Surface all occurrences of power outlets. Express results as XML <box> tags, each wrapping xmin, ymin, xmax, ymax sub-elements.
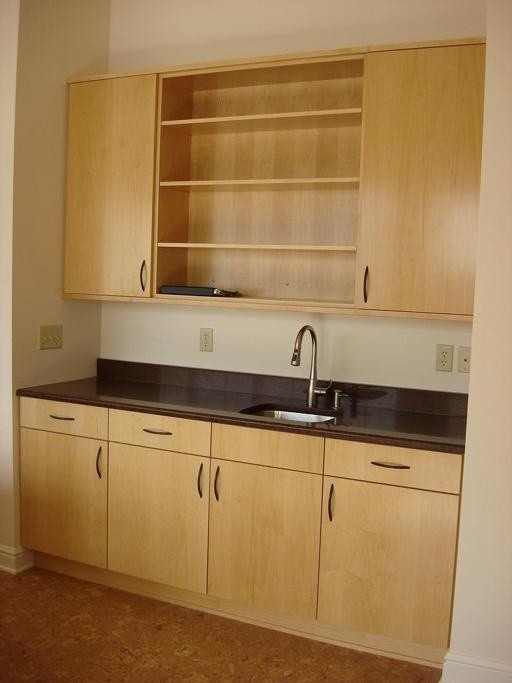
<box><xmin>199</xmin><ymin>327</ymin><xmax>213</xmax><ymax>352</ymax></box>
<box><xmin>435</xmin><ymin>343</ymin><xmax>454</xmax><ymax>372</ymax></box>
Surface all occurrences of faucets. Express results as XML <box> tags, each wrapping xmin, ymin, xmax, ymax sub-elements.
<box><xmin>290</xmin><ymin>324</ymin><xmax>319</xmax><ymax>408</ymax></box>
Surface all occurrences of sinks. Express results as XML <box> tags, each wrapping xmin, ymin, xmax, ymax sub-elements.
<box><xmin>238</xmin><ymin>402</ymin><xmax>338</xmax><ymax>424</ymax></box>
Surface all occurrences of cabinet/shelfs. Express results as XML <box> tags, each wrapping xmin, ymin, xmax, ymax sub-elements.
<box><xmin>359</xmin><ymin>37</ymin><xmax>484</xmax><ymax>315</ymax></box>
<box><xmin>157</xmin><ymin>49</ymin><xmax>365</xmax><ymax>308</ymax></box>
<box><xmin>18</xmin><ymin>395</ymin><xmax>109</xmax><ymax>572</ymax></box>
<box><xmin>58</xmin><ymin>69</ymin><xmax>192</xmax><ymax>304</ymax></box>
<box><xmin>107</xmin><ymin>407</ymin><xmax>326</xmax><ymax>629</ymax></box>
<box><xmin>315</xmin><ymin>434</ymin><xmax>464</xmax><ymax>651</ymax></box>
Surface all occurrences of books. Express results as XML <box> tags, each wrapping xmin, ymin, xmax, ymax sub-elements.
<box><xmin>160</xmin><ymin>285</ymin><xmax>240</xmax><ymax>296</ymax></box>
